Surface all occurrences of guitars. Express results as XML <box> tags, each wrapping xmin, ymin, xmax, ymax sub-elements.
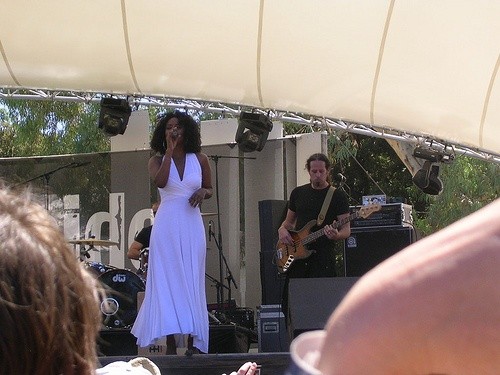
<box><xmin>275</xmin><ymin>199</ymin><xmax>383</xmax><ymax>274</ymax></box>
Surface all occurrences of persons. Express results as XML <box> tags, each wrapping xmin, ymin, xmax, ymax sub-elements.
<box><xmin>131</xmin><ymin>110</ymin><xmax>214</xmax><ymax>353</ymax></box>
<box><xmin>222</xmin><ymin>362</ymin><xmax>257</xmax><ymax>375</ymax></box>
<box><xmin>290</xmin><ymin>200</ymin><xmax>500</xmax><ymax>375</ymax></box>
<box><xmin>278</xmin><ymin>153</ymin><xmax>350</xmax><ymax>321</ymax></box>
<box><xmin>0</xmin><ymin>190</ymin><xmax>160</xmax><ymax>375</ymax></box>
<box><xmin>127</xmin><ymin>200</ymin><xmax>161</xmax><ymax>262</ymax></box>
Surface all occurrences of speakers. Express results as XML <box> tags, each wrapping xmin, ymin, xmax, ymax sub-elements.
<box><xmin>208</xmin><ymin>324</ymin><xmax>253</xmax><ymax>355</ymax></box>
<box><xmin>95</xmin><ymin>329</ymin><xmax>138</xmax><ymax>357</ymax></box>
<box><xmin>344</xmin><ymin>226</ymin><xmax>420</xmax><ymax>280</ymax></box>
<box><xmin>286</xmin><ymin>277</ymin><xmax>360</xmax><ymax>338</ymax></box>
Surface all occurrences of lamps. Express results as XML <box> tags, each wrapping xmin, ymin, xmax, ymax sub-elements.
<box><xmin>236</xmin><ymin>111</ymin><xmax>273</xmax><ymax>153</ymax></box>
<box><xmin>98</xmin><ymin>98</ymin><xmax>132</xmax><ymax>137</ymax></box>
<box><xmin>413</xmin><ymin>148</ymin><xmax>454</xmax><ymax>195</ymax></box>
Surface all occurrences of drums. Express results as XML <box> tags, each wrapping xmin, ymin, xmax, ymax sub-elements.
<box><xmin>139</xmin><ymin>247</ymin><xmax>149</xmax><ymax>276</ymax></box>
<box><xmin>91</xmin><ymin>266</ymin><xmax>145</xmax><ymax>330</ymax></box>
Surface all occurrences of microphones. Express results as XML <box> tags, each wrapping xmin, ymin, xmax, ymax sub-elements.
<box><xmin>209</xmin><ymin>221</ymin><xmax>212</xmax><ymax>242</ymax></box>
<box><xmin>169</xmin><ymin>129</ymin><xmax>178</xmax><ymax>140</ymax></box>
<box><xmin>70</xmin><ymin>161</ymin><xmax>92</xmax><ymax>168</ymax></box>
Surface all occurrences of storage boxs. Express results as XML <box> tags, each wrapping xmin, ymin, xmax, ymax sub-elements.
<box><xmin>256</xmin><ymin>304</ymin><xmax>290</xmax><ymax>353</ymax></box>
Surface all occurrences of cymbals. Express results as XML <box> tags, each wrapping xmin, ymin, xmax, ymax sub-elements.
<box><xmin>201</xmin><ymin>212</ymin><xmax>223</xmax><ymax>216</ymax></box>
<box><xmin>67</xmin><ymin>239</ymin><xmax>119</xmax><ymax>246</ymax></box>
